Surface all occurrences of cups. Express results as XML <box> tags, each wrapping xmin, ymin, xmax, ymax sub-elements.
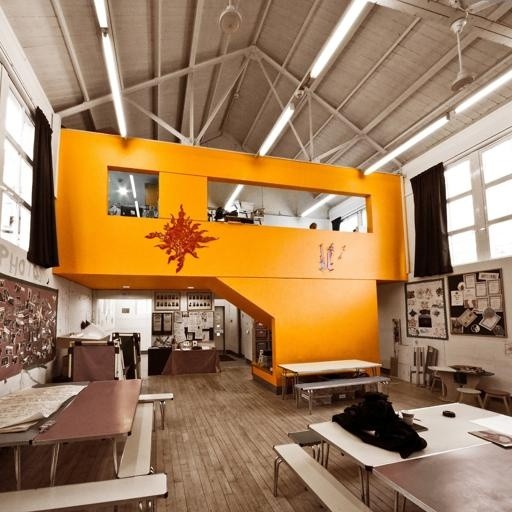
<box><xmin>402</xmin><ymin>410</ymin><xmax>415</xmax><ymax>427</ymax></box>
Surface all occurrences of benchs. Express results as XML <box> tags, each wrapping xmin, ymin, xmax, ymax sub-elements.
<box><xmin>274</xmin><ymin>427</ymin><xmax>378</xmax><ymax>512</ymax></box>
<box><xmin>0</xmin><ymin>393</ymin><xmax>173</xmax><ymax>511</ymax></box>
<box><xmin>279</xmin><ymin>369</ymin><xmax>392</xmax><ymax>415</ymax></box>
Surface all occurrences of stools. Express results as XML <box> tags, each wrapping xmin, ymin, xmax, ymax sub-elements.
<box><xmin>455</xmin><ymin>388</ymin><xmax>510</xmax><ymax>413</ymax></box>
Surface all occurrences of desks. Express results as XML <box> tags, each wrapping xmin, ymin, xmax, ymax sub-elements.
<box><xmin>276</xmin><ymin>358</ymin><xmax>383</xmax><ymax>400</ymax></box>
<box><xmin>305</xmin><ymin>400</ymin><xmax>512</xmax><ymax>512</ymax></box>
<box><xmin>428</xmin><ymin>365</ymin><xmax>484</xmax><ymax>402</ymax></box>
<box><xmin>0</xmin><ymin>378</ymin><xmax>144</xmax><ymax>511</ymax></box>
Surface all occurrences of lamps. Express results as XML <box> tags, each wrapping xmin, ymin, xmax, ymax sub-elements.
<box><xmin>309</xmin><ymin>0</ymin><xmax>371</xmax><ymax>79</ymax></box>
<box><xmin>299</xmin><ymin>194</ymin><xmax>337</xmax><ymax>218</ymax></box>
<box><xmin>454</xmin><ymin>69</ymin><xmax>511</xmax><ymax>114</ymax></box>
<box><xmin>220</xmin><ymin>0</ymin><xmax>242</xmax><ymax>35</ymax></box>
<box><xmin>223</xmin><ymin>184</ymin><xmax>245</xmax><ymax>212</ymax></box>
<box><xmin>448</xmin><ymin>13</ymin><xmax>475</xmax><ymax>93</ymax></box>
<box><xmin>257</xmin><ymin>102</ymin><xmax>296</xmax><ymax>157</ymax></box>
<box><xmin>363</xmin><ymin>112</ymin><xmax>451</xmax><ymax>178</ymax></box>
<box><xmin>92</xmin><ymin>0</ymin><xmax>142</xmax><ymax>220</ymax></box>
